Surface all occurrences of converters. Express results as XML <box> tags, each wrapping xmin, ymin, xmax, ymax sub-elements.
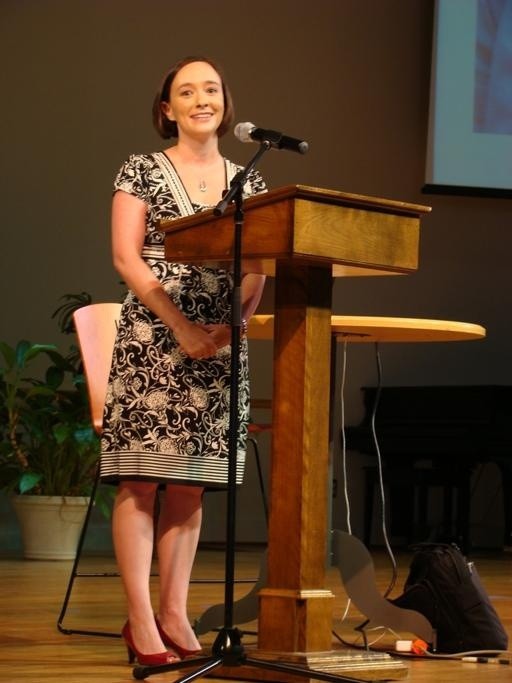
<box><xmin>395</xmin><ymin>641</ymin><xmax>412</xmax><ymax>652</ymax></box>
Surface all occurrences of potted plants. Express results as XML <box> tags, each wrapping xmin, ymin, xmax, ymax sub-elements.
<box><xmin>1</xmin><ymin>293</ymin><xmax>119</xmax><ymax>564</ymax></box>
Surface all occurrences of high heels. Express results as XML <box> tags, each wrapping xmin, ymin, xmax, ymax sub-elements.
<box><xmin>122</xmin><ymin>614</ymin><xmax>208</xmax><ymax>666</ymax></box>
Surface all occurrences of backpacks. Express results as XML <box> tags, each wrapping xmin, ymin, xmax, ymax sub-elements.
<box><xmin>386</xmin><ymin>542</ymin><xmax>509</xmax><ymax>657</ymax></box>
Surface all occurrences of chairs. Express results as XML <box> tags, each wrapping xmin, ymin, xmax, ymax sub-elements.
<box><xmin>56</xmin><ymin>301</ymin><xmax>270</xmax><ymax>638</ymax></box>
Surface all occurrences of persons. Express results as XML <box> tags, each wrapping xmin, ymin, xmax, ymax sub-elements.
<box><xmin>102</xmin><ymin>58</ymin><xmax>271</xmax><ymax>665</ymax></box>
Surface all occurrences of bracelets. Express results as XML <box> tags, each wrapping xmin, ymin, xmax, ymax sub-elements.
<box><xmin>241</xmin><ymin>319</ymin><xmax>248</xmax><ymax>334</ymax></box>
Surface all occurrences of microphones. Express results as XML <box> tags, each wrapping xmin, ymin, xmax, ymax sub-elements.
<box><xmin>233</xmin><ymin>121</ymin><xmax>309</xmax><ymax>156</ymax></box>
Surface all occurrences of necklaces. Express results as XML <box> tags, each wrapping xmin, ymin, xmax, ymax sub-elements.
<box><xmin>199</xmin><ymin>178</ymin><xmax>208</xmax><ymax>192</ymax></box>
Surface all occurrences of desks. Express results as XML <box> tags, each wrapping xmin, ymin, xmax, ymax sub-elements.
<box><xmin>245</xmin><ymin>314</ymin><xmax>488</xmax><ymax>651</ymax></box>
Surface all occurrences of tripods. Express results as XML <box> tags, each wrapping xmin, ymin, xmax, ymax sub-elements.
<box><xmin>132</xmin><ymin>139</ymin><xmax>377</xmax><ymax>683</ymax></box>
